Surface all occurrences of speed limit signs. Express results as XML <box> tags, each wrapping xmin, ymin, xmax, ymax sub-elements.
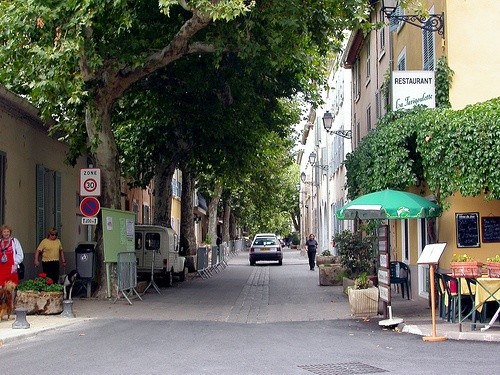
<box><xmin>84</xmin><ymin>178</ymin><xmax>98</xmax><ymax>193</ymax></box>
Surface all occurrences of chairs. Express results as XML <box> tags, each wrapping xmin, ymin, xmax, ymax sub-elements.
<box><xmin>390</xmin><ymin>260</ymin><xmax>410</xmax><ymax>300</ymax></box>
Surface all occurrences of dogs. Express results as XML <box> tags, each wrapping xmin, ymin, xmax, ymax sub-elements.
<box><xmin>0</xmin><ymin>281</ymin><xmax>16</xmax><ymax>321</ymax></box>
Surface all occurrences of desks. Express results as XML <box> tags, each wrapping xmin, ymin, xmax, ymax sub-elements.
<box><xmin>438</xmin><ymin>273</ymin><xmax>500</xmax><ymax>330</ymax></box>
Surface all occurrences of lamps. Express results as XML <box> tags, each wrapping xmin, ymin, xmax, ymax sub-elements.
<box><xmin>292</xmin><ymin>195</ymin><xmax>303</xmax><ymax>204</ymax></box>
<box><xmin>296</xmin><ymin>182</ymin><xmax>307</xmax><ymax>195</ymax></box>
<box><xmin>309</xmin><ymin>152</ymin><xmax>327</xmax><ymax>171</ymax></box>
<box><xmin>381</xmin><ymin>0</ymin><xmax>444</xmax><ymax>38</ymax></box>
<box><xmin>322</xmin><ymin>111</ymin><xmax>351</xmax><ymax>139</ymax></box>
<box><xmin>301</xmin><ymin>172</ymin><xmax>313</xmax><ymax>186</ymax></box>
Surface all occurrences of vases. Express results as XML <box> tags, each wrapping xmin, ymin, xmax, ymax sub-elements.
<box><xmin>450</xmin><ymin>261</ymin><xmax>484</xmax><ymax>276</ymax></box>
<box><xmin>15</xmin><ymin>290</ymin><xmax>64</xmax><ymax>314</ymax></box>
<box><xmin>486</xmin><ymin>261</ymin><xmax>500</xmax><ymax>277</ymax></box>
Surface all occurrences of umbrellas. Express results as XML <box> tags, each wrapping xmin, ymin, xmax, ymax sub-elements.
<box><xmin>335</xmin><ymin>188</ymin><xmax>442</xmax><ymax>319</ymax></box>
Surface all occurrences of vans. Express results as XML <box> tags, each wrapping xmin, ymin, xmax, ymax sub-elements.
<box><xmin>254</xmin><ymin>233</ymin><xmax>276</xmax><ymax>237</ymax></box>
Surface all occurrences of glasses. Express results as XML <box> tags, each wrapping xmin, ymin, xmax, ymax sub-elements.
<box><xmin>50</xmin><ymin>233</ymin><xmax>58</xmax><ymax>237</ymax></box>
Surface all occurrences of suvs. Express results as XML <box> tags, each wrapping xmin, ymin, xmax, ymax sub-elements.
<box><xmin>136</xmin><ymin>224</ymin><xmax>187</xmax><ymax>286</ymax></box>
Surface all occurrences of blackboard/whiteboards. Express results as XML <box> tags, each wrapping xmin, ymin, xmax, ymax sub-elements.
<box><xmin>481</xmin><ymin>216</ymin><xmax>500</xmax><ymax>243</ymax></box>
<box><xmin>455</xmin><ymin>212</ymin><xmax>481</xmax><ymax>248</ymax></box>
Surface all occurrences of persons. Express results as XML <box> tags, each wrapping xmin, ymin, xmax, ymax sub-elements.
<box><xmin>34</xmin><ymin>227</ymin><xmax>66</xmax><ymax>284</ymax></box>
<box><xmin>217</xmin><ymin>235</ymin><xmax>222</xmax><ymax>250</ymax></box>
<box><xmin>0</xmin><ymin>225</ymin><xmax>24</xmax><ymax>286</ymax></box>
<box><xmin>305</xmin><ymin>234</ymin><xmax>318</xmax><ymax>269</ymax></box>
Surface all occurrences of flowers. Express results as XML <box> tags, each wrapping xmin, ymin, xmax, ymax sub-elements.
<box><xmin>18</xmin><ymin>273</ymin><xmax>63</xmax><ymax>292</ymax></box>
<box><xmin>452</xmin><ymin>253</ymin><xmax>477</xmax><ymax>262</ymax></box>
<box><xmin>487</xmin><ymin>254</ymin><xmax>500</xmax><ymax>263</ymax></box>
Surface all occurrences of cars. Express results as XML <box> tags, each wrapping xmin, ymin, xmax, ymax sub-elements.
<box><xmin>249</xmin><ymin>237</ymin><xmax>283</xmax><ymax>265</ymax></box>
<box><xmin>278</xmin><ymin>237</ymin><xmax>285</xmax><ymax>247</ymax></box>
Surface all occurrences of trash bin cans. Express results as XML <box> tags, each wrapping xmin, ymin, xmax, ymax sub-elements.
<box><xmin>196</xmin><ymin>247</ymin><xmax>208</xmax><ymax>270</ymax></box>
<box><xmin>74</xmin><ymin>241</ymin><xmax>99</xmax><ymax>301</ymax></box>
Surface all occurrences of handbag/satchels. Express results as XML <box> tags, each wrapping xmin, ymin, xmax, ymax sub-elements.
<box><xmin>17</xmin><ymin>262</ymin><xmax>24</xmax><ymax>279</ymax></box>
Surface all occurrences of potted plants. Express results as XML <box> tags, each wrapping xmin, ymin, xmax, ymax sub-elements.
<box><xmin>333</xmin><ymin>230</ymin><xmax>378</xmax><ymax>291</ymax></box>
<box><xmin>319</xmin><ymin>264</ymin><xmax>342</xmax><ymax>285</ymax></box>
<box><xmin>348</xmin><ymin>272</ymin><xmax>379</xmax><ymax>317</ymax></box>
<box><xmin>316</xmin><ymin>249</ymin><xmax>335</xmax><ymax>266</ymax></box>
